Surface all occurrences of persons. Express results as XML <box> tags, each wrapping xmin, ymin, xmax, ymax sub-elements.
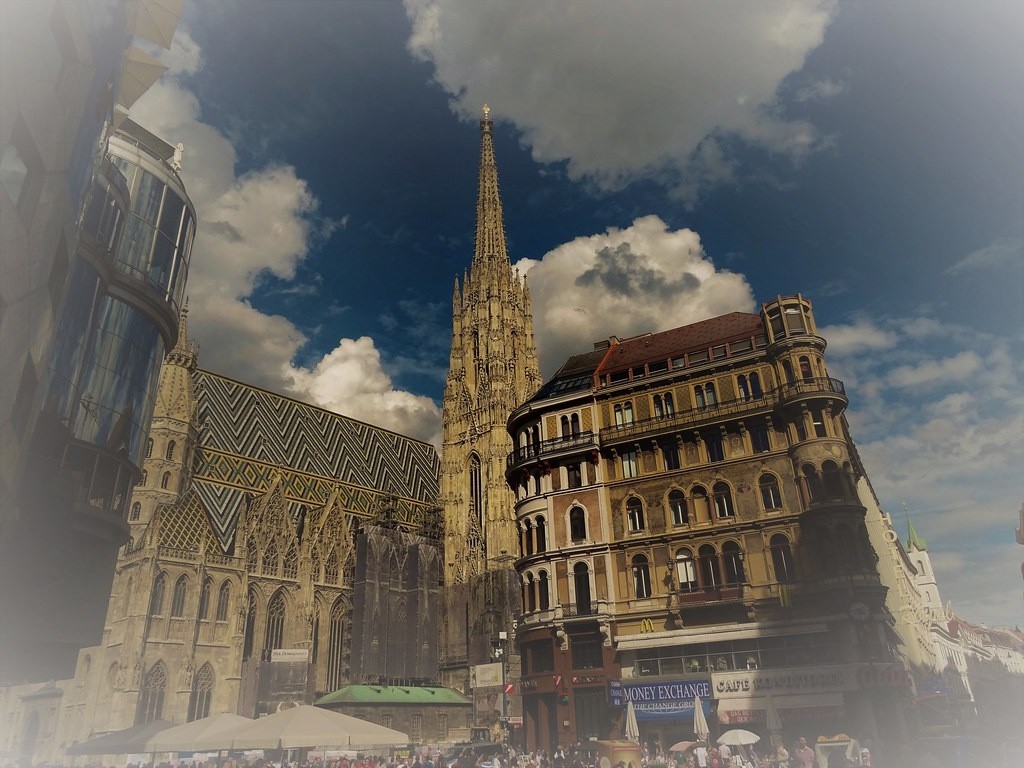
<box><xmin>480</xmin><ymin>694</ymin><xmax>501</xmax><ymax>725</ymax></box>
<box><xmin>93</xmin><ymin>753</ymin><xmax>447</xmax><ymax>768</ymax></box>
<box><xmin>453</xmin><ymin>730</ymin><xmax>873</xmax><ymax>768</ymax></box>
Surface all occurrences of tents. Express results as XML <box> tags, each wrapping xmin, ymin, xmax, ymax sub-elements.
<box><xmin>65</xmin><ymin>705</ymin><xmax>408</xmax><ymax>768</ymax></box>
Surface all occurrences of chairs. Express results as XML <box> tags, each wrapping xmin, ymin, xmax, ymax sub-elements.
<box><xmin>690</xmin><ymin>659</ymin><xmax>702</xmax><ymax>672</ymax></box>
<box><xmin>746</xmin><ymin>656</ymin><xmax>757</xmax><ymax>669</ymax></box>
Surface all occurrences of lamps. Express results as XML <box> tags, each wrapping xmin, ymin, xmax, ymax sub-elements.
<box><xmin>665</xmin><ymin>556</ymin><xmax>677</xmax><ymax>573</ymax></box>
<box><xmin>736</xmin><ymin>551</ymin><xmax>746</xmax><ymax>564</ymax></box>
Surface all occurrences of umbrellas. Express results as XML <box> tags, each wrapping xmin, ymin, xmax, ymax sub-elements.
<box><xmin>694</xmin><ymin>696</ymin><xmax>710</xmax><ymax>739</ymax></box>
<box><xmin>766</xmin><ymin>703</ymin><xmax>782</xmax><ymax>731</ymax></box>
<box><xmin>717</xmin><ymin>729</ymin><xmax>760</xmax><ymax>745</ymax></box>
<box><xmin>691</xmin><ymin>740</ymin><xmax>709</xmax><ymax>747</ymax></box>
<box><xmin>625</xmin><ymin>701</ymin><xmax>639</xmax><ymax>740</ymax></box>
<box><xmin>669</xmin><ymin>741</ymin><xmax>694</xmax><ymax>752</ymax></box>
